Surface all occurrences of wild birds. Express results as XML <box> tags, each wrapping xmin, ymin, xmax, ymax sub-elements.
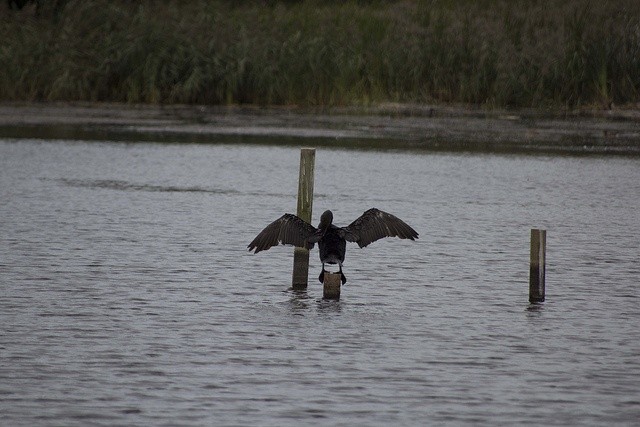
<box><xmin>246</xmin><ymin>207</ymin><xmax>420</xmax><ymax>286</ymax></box>
